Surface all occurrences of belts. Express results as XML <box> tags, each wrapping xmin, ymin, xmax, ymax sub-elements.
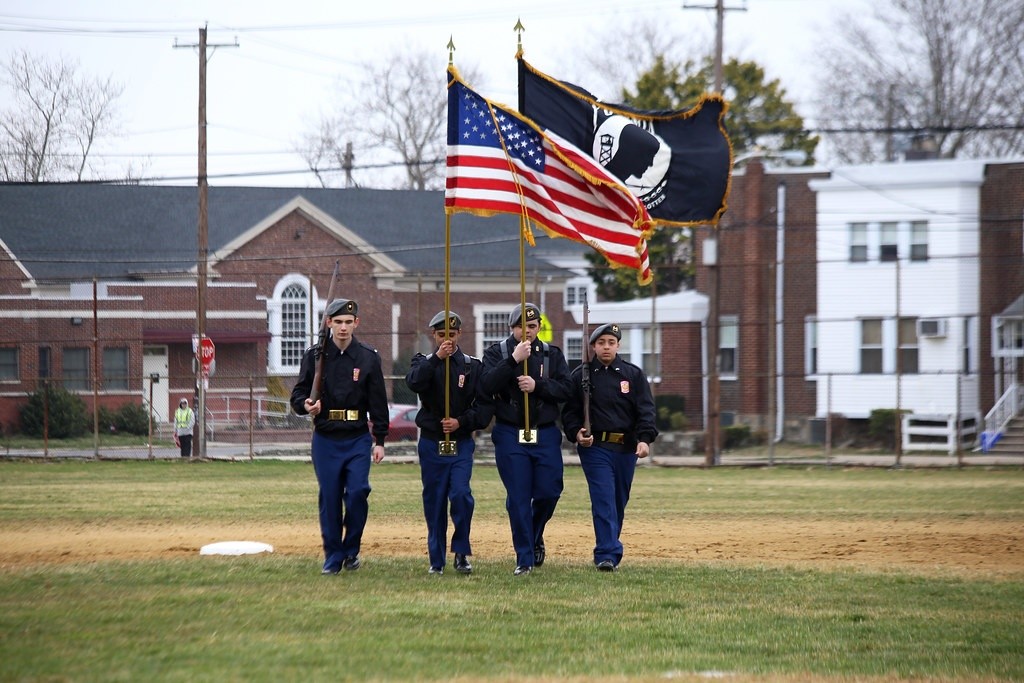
<box><xmin>495</xmin><ymin>417</ymin><xmax>556</xmax><ymax>429</ymax></box>
<box><xmin>420</xmin><ymin>429</ymin><xmax>472</xmax><ymax>442</ymax></box>
<box><xmin>315</xmin><ymin>410</ymin><xmax>367</xmax><ymax>421</ymax></box>
<box><xmin>590</xmin><ymin>432</ymin><xmax>633</xmax><ymax>443</ymax></box>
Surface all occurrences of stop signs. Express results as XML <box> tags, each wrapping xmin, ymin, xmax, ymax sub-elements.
<box><xmin>196</xmin><ymin>339</ymin><xmax>215</xmax><ymax>364</ymax></box>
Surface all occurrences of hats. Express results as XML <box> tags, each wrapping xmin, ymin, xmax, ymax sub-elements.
<box><xmin>323</xmin><ymin>298</ymin><xmax>358</xmax><ymax>317</ymax></box>
<box><xmin>429</xmin><ymin>309</ymin><xmax>461</xmax><ymax>331</ymax></box>
<box><xmin>507</xmin><ymin>303</ymin><xmax>540</xmax><ymax>327</ymax></box>
<box><xmin>589</xmin><ymin>323</ymin><xmax>621</xmax><ymax>345</ymax></box>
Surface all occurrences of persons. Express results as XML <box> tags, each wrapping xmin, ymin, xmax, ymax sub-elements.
<box><xmin>561</xmin><ymin>323</ymin><xmax>659</xmax><ymax>571</ymax></box>
<box><xmin>481</xmin><ymin>302</ymin><xmax>574</xmax><ymax>576</ymax></box>
<box><xmin>405</xmin><ymin>310</ymin><xmax>494</xmax><ymax>575</ymax></box>
<box><xmin>290</xmin><ymin>299</ymin><xmax>390</xmax><ymax>575</ymax></box>
<box><xmin>174</xmin><ymin>397</ymin><xmax>195</xmax><ymax>457</ymax></box>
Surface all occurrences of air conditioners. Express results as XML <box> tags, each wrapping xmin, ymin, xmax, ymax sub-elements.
<box><xmin>916</xmin><ymin>318</ymin><xmax>946</xmax><ymax>340</ymax></box>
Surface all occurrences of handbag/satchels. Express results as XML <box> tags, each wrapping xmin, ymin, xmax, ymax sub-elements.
<box><xmin>173</xmin><ymin>435</ymin><xmax>181</xmax><ymax>447</ymax></box>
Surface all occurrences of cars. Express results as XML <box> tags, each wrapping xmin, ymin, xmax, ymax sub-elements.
<box><xmin>367</xmin><ymin>403</ymin><xmax>419</xmax><ymax>451</ymax></box>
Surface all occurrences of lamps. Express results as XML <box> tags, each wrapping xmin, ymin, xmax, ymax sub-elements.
<box><xmin>71</xmin><ymin>316</ymin><xmax>84</xmax><ymax>325</ymax></box>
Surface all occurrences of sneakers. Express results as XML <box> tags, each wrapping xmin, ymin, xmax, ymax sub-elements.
<box><xmin>534</xmin><ymin>535</ymin><xmax>546</xmax><ymax>566</ymax></box>
<box><xmin>427</xmin><ymin>564</ymin><xmax>445</xmax><ymax>576</ymax></box>
<box><xmin>343</xmin><ymin>555</ymin><xmax>360</xmax><ymax>573</ymax></box>
<box><xmin>453</xmin><ymin>552</ymin><xmax>473</xmax><ymax>573</ymax></box>
<box><xmin>318</xmin><ymin>562</ymin><xmax>342</xmax><ymax>577</ymax></box>
<box><xmin>596</xmin><ymin>560</ymin><xmax>614</xmax><ymax>572</ymax></box>
<box><xmin>513</xmin><ymin>565</ymin><xmax>532</xmax><ymax>580</ymax></box>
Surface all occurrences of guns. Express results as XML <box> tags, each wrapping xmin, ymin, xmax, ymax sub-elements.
<box><xmin>307</xmin><ymin>256</ymin><xmax>341</xmax><ymax>406</ymax></box>
<box><xmin>582</xmin><ymin>290</ymin><xmax>593</xmax><ymax>439</ymax></box>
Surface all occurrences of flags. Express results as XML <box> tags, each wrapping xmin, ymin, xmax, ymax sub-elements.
<box><xmin>520</xmin><ymin>58</ymin><xmax>734</xmax><ymax>226</ymax></box>
<box><xmin>445</xmin><ymin>66</ymin><xmax>656</xmax><ymax>287</ymax></box>
